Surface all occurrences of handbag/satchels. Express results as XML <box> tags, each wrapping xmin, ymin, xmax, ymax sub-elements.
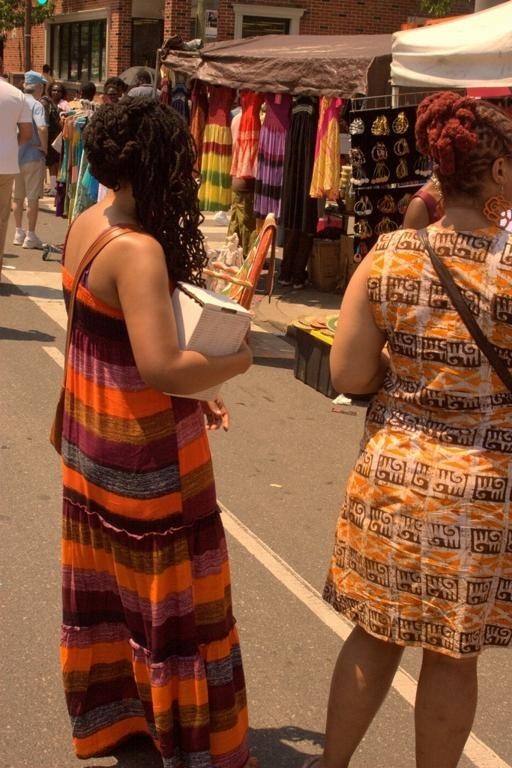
<box><xmin>50</xmin><ymin>385</ymin><xmax>65</xmax><ymax>455</ymax></box>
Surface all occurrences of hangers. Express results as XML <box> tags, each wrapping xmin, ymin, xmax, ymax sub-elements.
<box><xmin>56</xmin><ymin>98</ymin><xmax>101</xmax><ymax>127</ymax></box>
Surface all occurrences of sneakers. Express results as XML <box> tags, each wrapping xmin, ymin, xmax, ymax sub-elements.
<box><xmin>22</xmin><ymin>238</ymin><xmax>48</xmax><ymax>249</ymax></box>
<box><xmin>13</xmin><ymin>233</ymin><xmax>26</xmax><ymax>244</ymax></box>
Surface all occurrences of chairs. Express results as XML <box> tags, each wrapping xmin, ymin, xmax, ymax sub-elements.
<box><xmin>203</xmin><ymin>213</ymin><xmax>279</xmax><ymax>352</ymax></box>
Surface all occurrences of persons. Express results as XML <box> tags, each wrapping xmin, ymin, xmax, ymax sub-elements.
<box><xmin>403</xmin><ymin>169</ymin><xmax>444</xmax><ymax>227</ymax></box>
<box><xmin>52</xmin><ymin>95</ymin><xmax>258</xmax><ymax>767</ymax></box>
<box><xmin>303</xmin><ymin>90</ymin><xmax>510</xmax><ymax>768</ymax></box>
<box><xmin>0</xmin><ymin>59</ymin><xmax>133</xmax><ymax>261</ymax></box>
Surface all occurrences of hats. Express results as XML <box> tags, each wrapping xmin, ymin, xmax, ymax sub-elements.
<box><xmin>24</xmin><ymin>70</ymin><xmax>49</xmax><ymax>84</ymax></box>
